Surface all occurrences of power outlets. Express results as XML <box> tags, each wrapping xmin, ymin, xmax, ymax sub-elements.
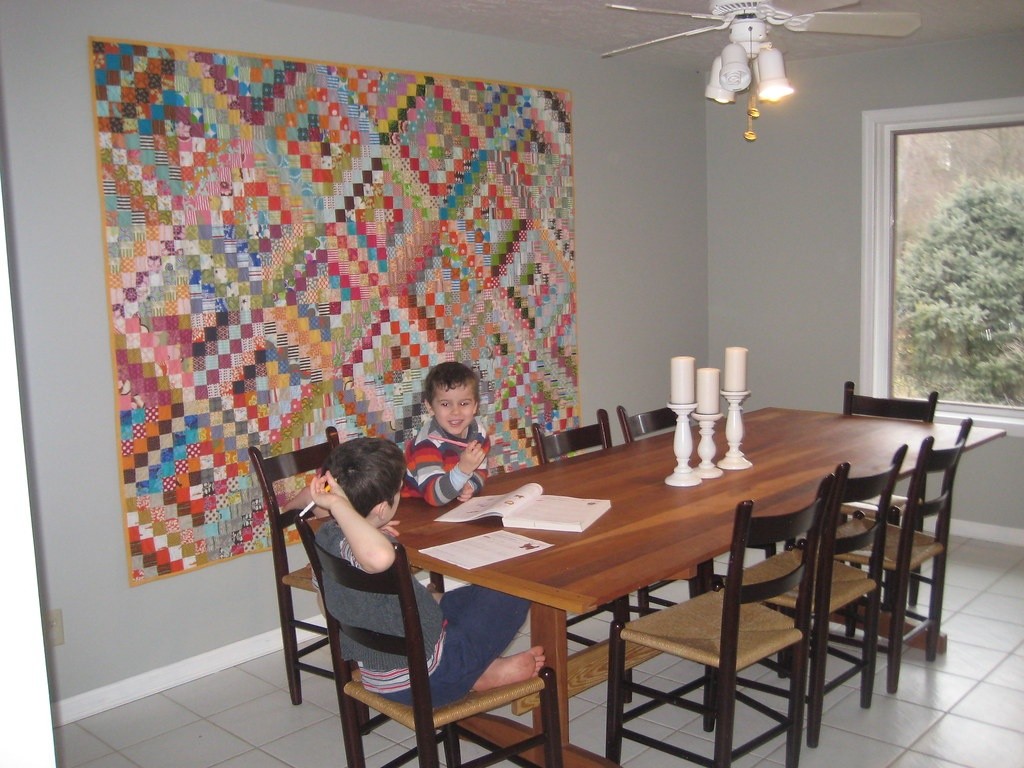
<box><xmin>42</xmin><ymin>610</ymin><xmax>65</xmax><ymax>649</ymax></box>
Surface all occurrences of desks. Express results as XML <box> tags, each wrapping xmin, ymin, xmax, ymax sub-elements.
<box><xmin>305</xmin><ymin>407</ymin><xmax>1007</xmax><ymax>767</ymax></box>
<box><xmin>600</xmin><ymin>1</ymin><xmax>922</xmax><ymax>62</ymax></box>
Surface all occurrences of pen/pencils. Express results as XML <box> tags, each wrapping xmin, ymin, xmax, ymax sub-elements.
<box><xmin>299</xmin><ymin>479</ymin><xmax>338</xmax><ymax>517</ymax></box>
<box><xmin>429</xmin><ymin>434</ymin><xmax>469</xmax><ymax>447</ymax></box>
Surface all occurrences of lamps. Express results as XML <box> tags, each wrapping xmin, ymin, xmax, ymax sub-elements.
<box><xmin>705</xmin><ymin>21</ymin><xmax>801</xmax><ymax>141</ymax></box>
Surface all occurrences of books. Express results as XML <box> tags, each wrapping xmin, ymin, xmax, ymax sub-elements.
<box><xmin>432</xmin><ymin>483</ymin><xmax>611</xmax><ymax>533</ymax></box>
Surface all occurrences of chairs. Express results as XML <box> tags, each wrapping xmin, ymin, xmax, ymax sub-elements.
<box><xmin>603</xmin><ymin>475</ymin><xmax>838</xmax><ymax>767</ymax></box>
<box><xmin>304</xmin><ymin>503</ymin><xmax>565</xmax><ymax>768</ymax></box>
<box><xmin>837</xmin><ymin>383</ymin><xmax>941</xmax><ymax>634</ymax></box>
<box><xmin>248</xmin><ymin>425</ymin><xmax>444</xmax><ymax>735</ymax></box>
<box><xmin>613</xmin><ymin>397</ymin><xmax>780</xmax><ymax>594</ymax></box>
<box><xmin>530</xmin><ymin>409</ymin><xmax>704</xmax><ymax>704</ymax></box>
<box><xmin>735</xmin><ymin>442</ymin><xmax>906</xmax><ymax>750</ymax></box>
<box><xmin>789</xmin><ymin>418</ymin><xmax>975</xmax><ymax>696</ymax></box>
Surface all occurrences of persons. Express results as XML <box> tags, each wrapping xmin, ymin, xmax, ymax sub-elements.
<box><xmin>398</xmin><ymin>362</ymin><xmax>490</xmax><ymax>507</ymax></box>
<box><xmin>282</xmin><ymin>436</ymin><xmax>546</xmax><ymax>710</ymax></box>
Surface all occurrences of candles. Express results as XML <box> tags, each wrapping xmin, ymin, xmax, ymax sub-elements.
<box><xmin>697</xmin><ymin>366</ymin><xmax>720</xmax><ymax>416</ymax></box>
<box><xmin>724</xmin><ymin>346</ymin><xmax>749</xmax><ymax>392</ymax></box>
<box><xmin>668</xmin><ymin>356</ymin><xmax>694</xmax><ymax>405</ymax></box>
